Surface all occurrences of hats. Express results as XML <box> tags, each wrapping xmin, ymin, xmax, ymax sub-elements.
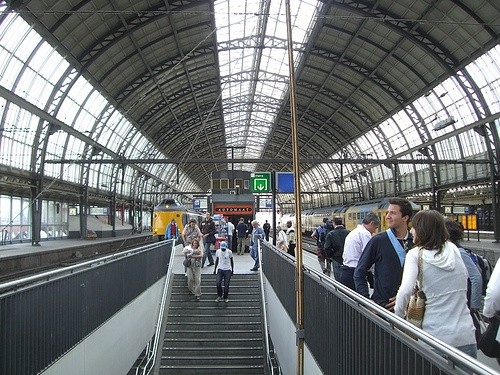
<box><xmin>220</xmin><ymin>241</ymin><xmax>227</xmax><ymax>248</ymax></box>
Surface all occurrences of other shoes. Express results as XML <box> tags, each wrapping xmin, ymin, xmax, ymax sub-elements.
<box><xmin>225</xmin><ymin>299</ymin><xmax>228</xmax><ymax>302</ymax></box>
<box><xmin>197</xmin><ymin>296</ymin><xmax>200</xmax><ymax>299</ymax></box>
<box><xmin>250</xmin><ymin>268</ymin><xmax>258</xmax><ymax>271</ymax></box>
<box><xmin>216</xmin><ymin>297</ymin><xmax>221</xmax><ymax>300</ymax></box>
<box><xmin>323</xmin><ymin>268</ymin><xmax>327</xmax><ymax>274</ymax></box>
<box><xmin>207</xmin><ymin>263</ymin><xmax>214</xmax><ymax>266</ymax></box>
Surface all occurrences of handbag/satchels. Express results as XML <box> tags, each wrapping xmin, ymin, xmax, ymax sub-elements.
<box><xmin>406</xmin><ymin>291</ymin><xmax>426</xmax><ymax>328</ymax></box>
<box><xmin>327</xmin><ymin>247</ymin><xmax>335</xmax><ymax>258</ymax></box>
<box><xmin>211</xmin><ymin>235</ymin><xmax>216</xmax><ymax>245</ymax></box>
<box><xmin>183</xmin><ymin>258</ymin><xmax>190</xmax><ymax>267</ymax></box>
<box><xmin>479</xmin><ymin>310</ymin><xmax>500</xmax><ymax>358</ymax></box>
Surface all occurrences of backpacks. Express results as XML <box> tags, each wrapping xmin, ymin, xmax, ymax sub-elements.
<box><xmin>465</xmin><ymin>248</ymin><xmax>493</xmax><ymax>296</ymax></box>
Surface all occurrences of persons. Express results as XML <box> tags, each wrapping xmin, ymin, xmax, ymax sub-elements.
<box><xmin>277</xmin><ymin>226</ymin><xmax>289</xmax><ymax>253</ymax></box>
<box><xmin>340</xmin><ymin>211</ymin><xmax>381</xmax><ymax>293</ymax></box>
<box><xmin>263</xmin><ymin>219</ymin><xmax>270</xmax><ymax>242</ymax></box>
<box><xmin>286</xmin><ymin>220</ymin><xmax>296</xmax><ymax>257</ymax></box>
<box><xmin>200</xmin><ymin>213</ymin><xmax>217</xmax><ymax>269</ymax></box>
<box><xmin>226</xmin><ymin>216</ymin><xmax>235</xmax><ymax>251</ymax></box>
<box><xmin>483</xmin><ymin>208</ymin><xmax>500</xmax><ymax>366</ymax></box>
<box><xmin>442</xmin><ymin>217</ymin><xmax>482</xmax><ymax>350</ymax></box>
<box><xmin>182</xmin><ymin>219</ymin><xmax>202</xmax><ymax>277</ymax></box>
<box><xmin>394</xmin><ymin>210</ymin><xmax>476</xmax><ymax>361</ymax></box>
<box><xmin>213</xmin><ymin>241</ymin><xmax>234</xmax><ymax>303</ymax></box>
<box><xmin>354</xmin><ymin>198</ymin><xmax>414</xmax><ymax>315</ymax></box>
<box><xmin>182</xmin><ymin>239</ymin><xmax>202</xmax><ymax>301</ymax></box>
<box><xmin>277</xmin><ymin>222</ymin><xmax>282</xmax><ymax>229</ymax></box>
<box><xmin>236</xmin><ymin>217</ymin><xmax>251</xmax><ymax>256</ymax></box>
<box><xmin>249</xmin><ymin>220</ymin><xmax>264</xmax><ymax>271</ymax></box>
<box><xmin>311</xmin><ymin>218</ymin><xmax>331</xmax><ymax>278</ymax></box>
<box><xmin>164</xmin><ymin>217</ymin><xmax>180</xmax><ymax>246</ymax></box>
<box><xmin>324</xmin><ymin>215</ymin><xmax>351</xmax><ymax>282</ymax></box>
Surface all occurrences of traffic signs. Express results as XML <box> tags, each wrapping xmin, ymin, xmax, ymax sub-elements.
<box><xmin>249</xmin><ymin>172</ymin><xmax>271</xmax><ymax>193</ymax></box>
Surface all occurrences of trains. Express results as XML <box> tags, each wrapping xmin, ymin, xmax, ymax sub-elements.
<box><xmin>152</xmin><ymin>198</ymin><xmax>207</xmax><ymax>241</ymax></box>
<box><xmin>279</xmin><ymin>197</ymin><xmax>421</xmax><ymax>237</ymax></box>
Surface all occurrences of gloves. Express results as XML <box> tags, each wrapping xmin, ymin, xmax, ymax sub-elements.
<box><xmin>471</xmin><ymin>308</ymin><xmax>479</xmax><ymax>319</ymax></box>
